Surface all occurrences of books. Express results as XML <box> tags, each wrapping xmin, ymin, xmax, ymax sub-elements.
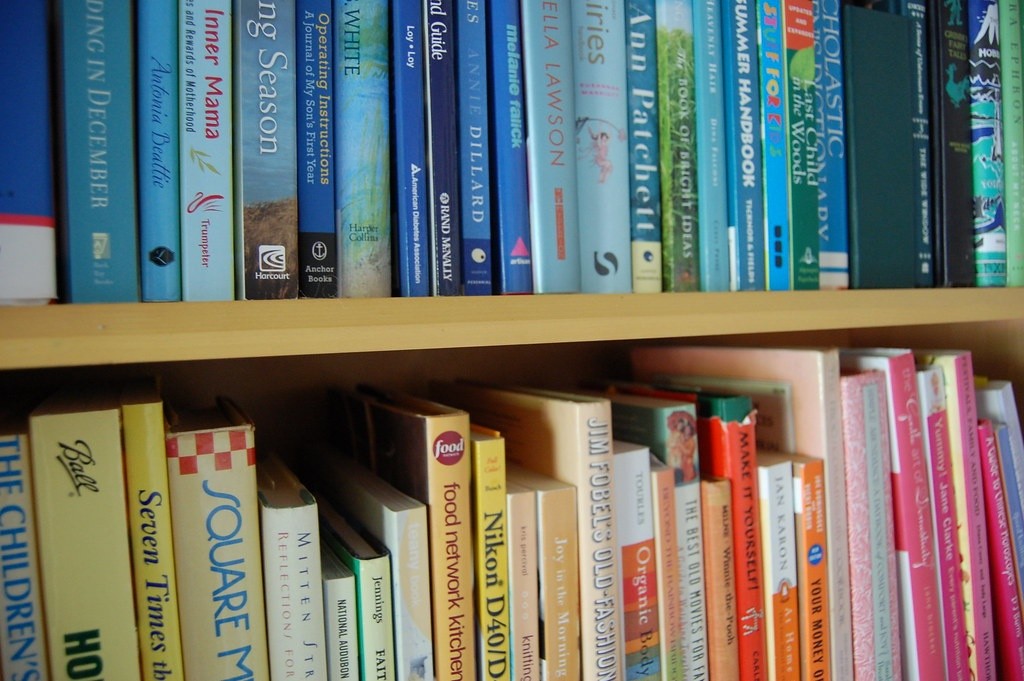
<box><xmin>0</xmin><ymin>341</ymin><xmax>1024</xmax><ymax>681</ymax></box>
<box><xmin>0</xmin><ymin>0</ymin><xmax>1024</xmax><ymax>303</ymax></box>
